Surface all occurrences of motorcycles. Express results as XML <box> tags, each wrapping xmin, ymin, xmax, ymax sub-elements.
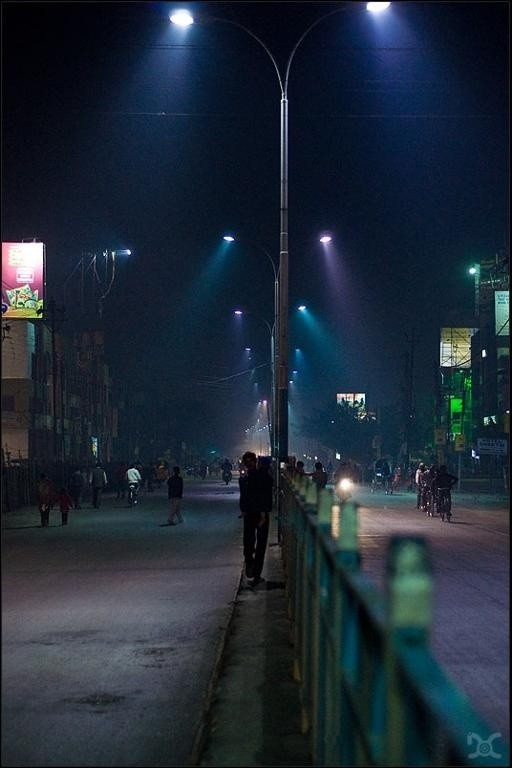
<box><xmin>201</xmin><ymin>469</ymin><xmax>248</xmax><ymax>485</ymax></box>
<box><xmin>335</xmin><ymin>478</ymin><xmax>354</xmax><ymax>503</ymax></box>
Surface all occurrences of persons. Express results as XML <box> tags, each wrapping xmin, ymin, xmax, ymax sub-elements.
<box><xmin>167</xmin><ymin>466</ymin><xmax>184</xmax><ymax>526</ymax></box>
<box><xmin>54</xmin><ymin>487</ymin><xmax>74</xmax><ymax>527</ymax></box>
<box><xmin>312</xmin><ymin>462</ymin><xmax>328</xmax><ymax>491</ymax></box>
<box><xmin>71</xmin><ymin>460</ymin><xmax>167</xmax><ymax>510</ymax></box>
<box><xmin>366</xmin><ymin>456</ymin><xmax>459</xmax><ymax>517</ymax></box>
<box><xmin>37</xmin><ymin>472</ymin><xmax>53</xmax><ymax>528</ymax></box>
<box><xmin>191</xmin><ymin>456</ymin><xmax>234</xmax><ymax>485</ymax></box>
<box><xmin>294</xmin><ymin>461</ymin><xmax>309</xmax><ymax>479</ymax></box>
<box><xmin>238</xmin><ymin>451</ymin><xmax>272</xmax><ymax>586</ymax></box>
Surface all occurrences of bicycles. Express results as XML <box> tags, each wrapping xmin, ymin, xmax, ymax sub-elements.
<box><xmin>127</xmin><ymin>484</ymin><xmax>141</xmax><ymax>509</ymax></box>
<box><xmin>372</xmin><ymin>475</ymin><xmax>452</xmax><ymax>523</ymax></box>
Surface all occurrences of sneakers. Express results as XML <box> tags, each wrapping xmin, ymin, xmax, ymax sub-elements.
<box><xmin>168</xmin><ymin>519</ymin><xmax>185</xmax><ymax>525</ymax></box>
<box><xmin>243</xmin><ymin>574</ymin><xmax>265</xmax><ymax>587</ymax></box>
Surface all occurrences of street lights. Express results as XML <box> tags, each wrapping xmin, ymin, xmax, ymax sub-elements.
<box><xmin>168</xmin><ymin>1</ymin><xmax>396</xmax><ymax>463</ymax></box>
